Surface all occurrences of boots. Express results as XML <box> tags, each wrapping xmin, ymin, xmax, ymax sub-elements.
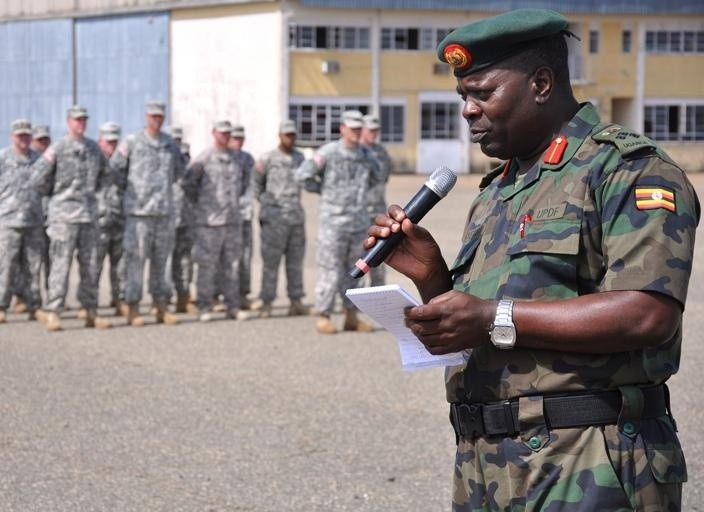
<box><xmin>211</xmin><ymin>292</ymin><xmax>270</xmax><ymax>321</ymax></box>
<box><xmin>1</xmin><ymin>297</ymin><xmax>70</xmax><ymax>330</ymax></box>
<box><xmin>151</xmin><ymin>293</ymin><xmax>212</xmax><ymax>323</ymax></box>
<box><xmin>289</xmin><ymin>300</ymin><xmax>310</xmax><ymax>314</ymax></box>
<box><xmin>78</xmin><ymin>298</ymin><xmax>145</xmax><ymax>328</ymax></box>
<box><xmin>316</xmin><ymin>313</ymin><xmax>337</xmax><ymax>333</ymax></box>
<box><xmin>343</xmin><ymin>308</ymin><xmax>372</xmax><ymax>332</ymax></box>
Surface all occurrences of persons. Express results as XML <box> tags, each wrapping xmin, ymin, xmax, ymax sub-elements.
<box><xmin>359</xmin><ymin>6</ymin><xmax>700</xmax><ymax>511</ymax></box>
<box><xmin>350</xmin><ymin>113</ymin><xmax>393</xmax><ymax>289</ymax></box>
<box><xmin>291</xmin><ymin>109</ymin><xmax>387</xmax><ymax>337</ymax></box>
<box><xmin>0</xmin><ymin>100</ymin><xmax>259</xmax><ymax>331</ymax></box>
<box><xmin>248</xmin><ymin>115</ymin><xmax>315</xmax><ymax>317</ymax></box>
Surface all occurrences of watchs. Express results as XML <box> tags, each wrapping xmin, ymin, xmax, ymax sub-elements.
<box><xmin>484</xmin><ymin>293</ymin><xmax>519</xmax><ymax>353</ymax></box>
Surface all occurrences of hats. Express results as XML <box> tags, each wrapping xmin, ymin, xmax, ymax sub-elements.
<box><xmin>146</xmin><ymin>100</ymin><xmax>166</xmax><ymax>116</ymax></box>
<box><xmin>229</xmin><ymin>126</ymin><xmax>244</xmax><ymax>138</ymax></box>
<box><xmin>11</xmin><ymin>105</ymin><xmax>89</xmax><ymax>142</ymax></box>
<box><xmin>180</xmin><ymin>142</ymin><xmax>190</xmax><ymax>156</ymax></box>
<box><xmin>278</xmin><ymin>119</ymin><xmax>297</xmax><ymax>134</ymax></box>
<box><xmin>212</xmin><ymin>121</ymin><xmax>231</xmax><ymax>133</ymax></box>
<box><xmin>340</xmin><ymin>110</ymin><xmax>363</xmax><ymax>128</ymax></box>
<box><xmin>437</xmin><ymin>9</ymin><xmax>582</xmax><ymax>77</ymax></box>
<box><xmin>164</xmin><ymin>125</ymin><xmax>183</xmax><ymax>139</ymax></box>
<box><xmin>99</xmin><ymin>121</ymin><xmax>121</xmax><ymax>142</ymax></box>
<box><xmin>362</xmin><ymin>114</ymin><xmax>382</xmax><ymax>130</ymax></box>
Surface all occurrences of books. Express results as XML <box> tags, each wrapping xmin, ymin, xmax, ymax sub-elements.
<box><xmin>343</xmin><ymin>282</ymin><xmax>469</xmax><ymax>372</ymax></box>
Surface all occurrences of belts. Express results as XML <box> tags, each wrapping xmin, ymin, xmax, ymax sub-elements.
<box><xmin>450</xmin><ymin>382</ymin><xmax>670</xmax><ymax>441</ymax></box>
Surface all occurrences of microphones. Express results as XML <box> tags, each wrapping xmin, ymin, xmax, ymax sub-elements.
<box><xmin>347</xmin><ymin>164</ymin><xmax>457</xmax><ymax>279</ymax></box>
